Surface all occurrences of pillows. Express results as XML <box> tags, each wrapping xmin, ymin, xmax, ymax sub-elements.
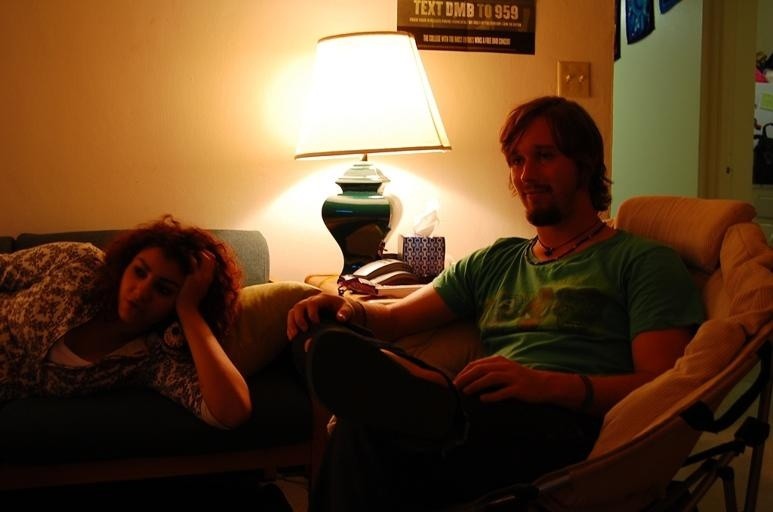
<box><xmin>219</xmin><ymin>281</ymin><xmax>328</xmax><ymax>381</ymax></box>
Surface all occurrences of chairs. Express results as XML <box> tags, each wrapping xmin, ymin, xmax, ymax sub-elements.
<box><xmin>392</xmin><ymin>195</ymin><xmax>773</xmax><ymax>512</ymax></box>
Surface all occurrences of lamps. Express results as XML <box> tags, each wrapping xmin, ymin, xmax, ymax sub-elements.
<box><xmin>294</xmin><ymin>30</ymin><xmax>454</xmax><ymax>283</ymax></box>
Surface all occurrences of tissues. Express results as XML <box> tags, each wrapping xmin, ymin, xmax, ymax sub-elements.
<box><xmin>399</xmin><ymin>210</ymin><xmax>446</xmax><ymax>279</ymax></box>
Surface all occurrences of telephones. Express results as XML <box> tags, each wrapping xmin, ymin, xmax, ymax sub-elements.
<box><xmin>353</xmin><ymin>257</ymin><xmax>419</xmax><ymax>284</ymax></box>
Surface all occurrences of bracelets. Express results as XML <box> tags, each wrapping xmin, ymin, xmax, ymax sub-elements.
<box><xmin>582</xmin><ymin>373</ymin><xmax>594</xmax><ymax>407</ymax></box>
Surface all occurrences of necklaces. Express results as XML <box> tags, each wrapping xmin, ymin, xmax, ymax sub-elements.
<box><xmin>538</xmin><ymin>217</ymin><xmax>602</xmax><ymax>256</ymax></box>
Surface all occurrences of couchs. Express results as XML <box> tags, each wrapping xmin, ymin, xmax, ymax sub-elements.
<box><xmin>1</xmin><ymin>227</ymin><xmax>338</xmax><ymax>512</ymax></box>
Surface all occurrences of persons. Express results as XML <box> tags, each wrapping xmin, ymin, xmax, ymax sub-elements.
<box><xmin>287</xmin><ymin>95</ymin><xmax>701</xmax><ymax>511</ymax></box>
<box><xmin>0</xmin><ymin>212</ymin><xmax>252</xmax><ymax>431</ymax></box>
